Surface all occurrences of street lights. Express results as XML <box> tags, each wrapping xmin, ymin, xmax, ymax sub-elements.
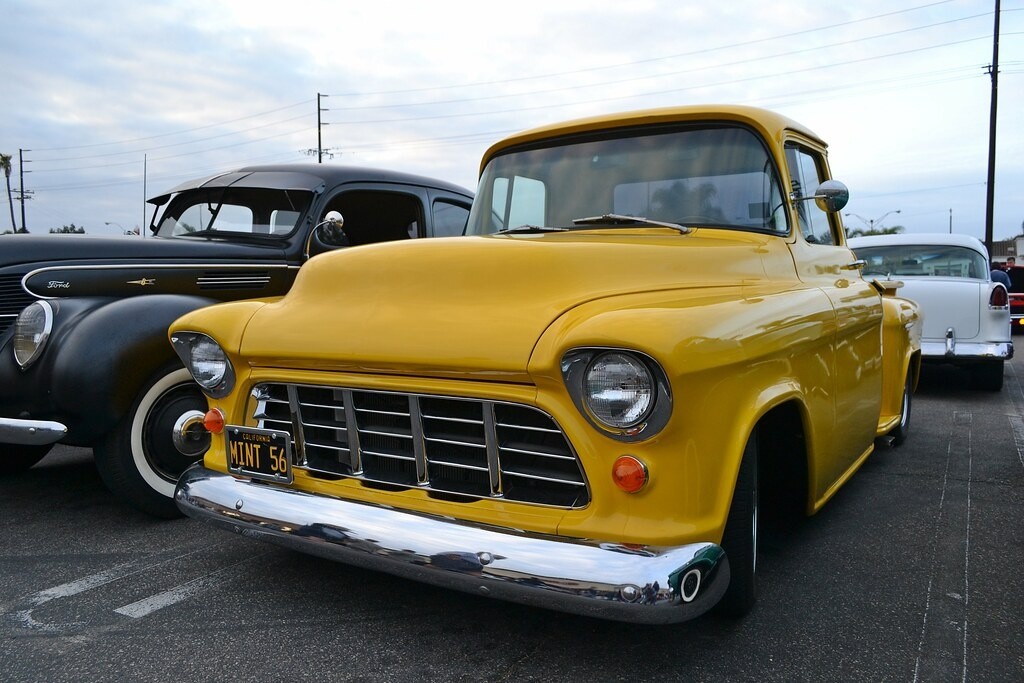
<box><xmin>844</xmin><ymin>210</ymin><xmax>902</xmax><ymax>236</ymax></box>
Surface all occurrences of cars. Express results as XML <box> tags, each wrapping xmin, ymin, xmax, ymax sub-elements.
<box><xmin>999</xmin><ymin>265</ymin><xmax>1024</xmax><ymax>331</ymax></box>
<box><xmin>845</xmin><ymin>232</ymin><xmax>1014</xmax><ymax>395</ymax></box>
<box><xmin>0</xmin><ymin>161</ymin><xmax>507</xmax><ymax>519</ymax></box>
<box><xmin>165</xmin><ymin>106</ymin><xmax>925</xmax><ymax>625</ymax></box>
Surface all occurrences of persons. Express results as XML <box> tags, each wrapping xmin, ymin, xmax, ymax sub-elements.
<box><xmin>990</xmin><ymin>256</ymin><xmax>1017</xmax><ymax>292</ymax></box>
